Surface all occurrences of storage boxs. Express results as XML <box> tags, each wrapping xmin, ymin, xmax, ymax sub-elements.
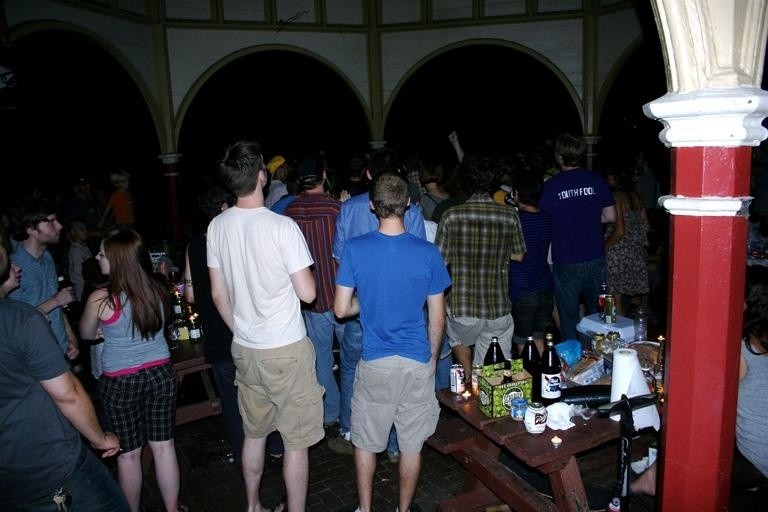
<box><xmin>575</xmin><ymin>313</ymin><xmax>643</xmax><ymax>370</ymax></box>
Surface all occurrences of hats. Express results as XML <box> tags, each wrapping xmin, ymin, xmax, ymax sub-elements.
<box><xmin>300</xmin><ymin>154</ymin><xmax>325</xmax><ymax>177</ymax></box>
<box><xmin>268</xmin><ymin>155</ymin><xmax>285</xmax><ymax>180</ymax></box>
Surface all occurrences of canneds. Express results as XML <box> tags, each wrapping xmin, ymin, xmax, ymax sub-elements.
<box><xmin>450</xmin><ymin>364</ymin><xmax>465</xmax><ymax>395</ymax></box>
<box><xmin>590</xmin><ymin>332</ymin><xmax>605</xmax><ymax>351</ymax></box>
<box><xmin>604</xmin><ymin>296</ymin><xmax>617</xmax><ymax>323</ymax></box>
<box><xmin>607</xmin><ymin>332</ymin><xmax>620</xmax><ymax>341</ymax></box>
<box><xmin>168</xmin><ymin>325</ymin><xmax>179</xmax><ymax>341</ymax></box>
<box><xmin>472</xmin><ymin>365</ymin><xmax>482</xmax><ymax>394</ymax></box>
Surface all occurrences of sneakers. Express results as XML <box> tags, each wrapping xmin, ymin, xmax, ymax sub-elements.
<box><xmin>328</xmin><ymin>435</ymin><xmax>354</xmax><ymax>454</ymax></box>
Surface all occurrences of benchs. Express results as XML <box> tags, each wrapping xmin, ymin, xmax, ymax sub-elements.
<box><xmin>425</xmin><ymin>417</ymin><xmax>483</xmax><ymax>457</ymax></box>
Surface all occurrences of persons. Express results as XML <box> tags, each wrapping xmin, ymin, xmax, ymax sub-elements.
<box><xmin>736</xmin><ymin>279</ymin><xmax>768</xmax><ymax>492</ymax></box>
<box><xmin>2</xmin><ymin>171</ymin><xmax>188</xmax><ymax>512</ymax></box>
<box><xmin>333</xmin><ymin>174</ymin><xmax>454</xmax><ymax>512</ymax></box>
<box><xmin>207</xmin><ymin>142</ymin><xmax>327</xmax><ymax>512</ymax></box>
<box><xmin>189</xmin><ymin>142</ymin><xmax>669</xmax><ymax>461</ymax></box>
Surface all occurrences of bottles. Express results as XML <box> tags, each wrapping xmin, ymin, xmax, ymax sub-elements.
<box><xmin>510</xmin><ymin>398</ymin><xmax>530</xmax><ymax>421</ymax></box>
<box><xmin>501</xmin><ymin>359</ymin><xmax>514</xmax><ymax>384</ymax></box>
<box><xmin>521</xmin><ymin>332</ymin><xmax>541</xmax><ymax>402</ymax></box>
<box><xmin>597</xmin><ymin>282</ymin><xmax>608</xmax><ymax>321</ymax></box>
<box><xmin>484</xmin><ymin>337</ymin><xmax>505</xmax><ymax>366</ymax></box>
<box><xmin>634</xmin><ymin>307</ymin><xmax>647</xmax><ymax>340</ymax></box>
<box><xmin>174</xmin><ymin>297</ymin><xmax>205</xmax><ymax>343</ymax></box>
<box><xmin>641</xmin><ymin>363</ymin><xmax>656</xmax><ymax>393</ymax></box>
<box><xmin>541</xmin><ymin>332</ymin><xmax>561</xmax><ymax>407</ymax></box>
<box><xmin>56</xmin><ymin>275</ymin><xmax>77</xmax><ymax>313</ymax></box>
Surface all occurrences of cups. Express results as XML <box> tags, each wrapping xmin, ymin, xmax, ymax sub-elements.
<box><xmin>168</xmin><ymin>325</ymin><xmax>179</xmax><ymax>349</ymax></box>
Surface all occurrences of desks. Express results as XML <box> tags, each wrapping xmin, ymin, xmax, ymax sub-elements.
<box><xmin>173</xmin><ymin>343</ymin><xmax>223</xmax><ymax>428</ymax></box>
<box><xmin>437</xmin><ymin>381</ymin><xmax>640</xmax><ymax>511</ymax></box>
